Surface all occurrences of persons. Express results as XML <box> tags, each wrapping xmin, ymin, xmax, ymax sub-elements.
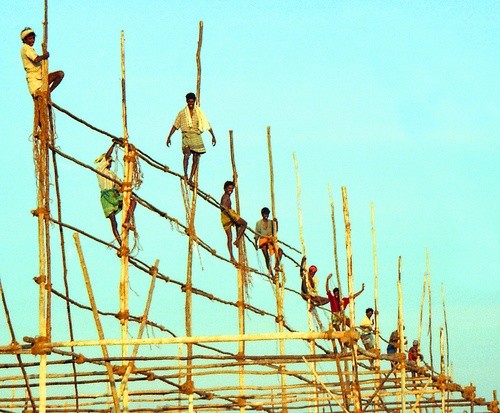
<box><xmin>254</xmin><ymin>207</ymin><xmax>285</xmax><ymax>281</ymax></box>
<box><xmin>408</xmin><ymin>338</ymin><xmax>423</xmax><ymax>380</ymax></box>
<box><xmin>325</xmin><ymin>273</ymin><xmax>364</xmax><ymax>352</ymax></box>
<box><xmin>94</xmin><ymin>139</ymin><xmax>137</xmax><ymax>246</ymax></box>
<box><xmin>361</xmin><ymin>308</ymin><xmax>380</xmax><ymax>370</ymax></box>
<box><xmin>20</xmin><ymin>26</ymin><xmax>64</xmax><ymax>138</ymax></box>
<box><xmin>221</xmin><ymin>181</ymin><xmax>248</xmax><ymax>264</ymax></box>
<box><xmin>300</xmin><ymin>256</ymin><xmax>329</xmax><ymax>311</ymax></box>
<box><xmin>166</xmin><ymin>94</ymin><xmax>216</xmax><ymax>186</ymax></box>
<box><xmin>387</xmin><ymin>325</ymin><xmax>407</xmax><ymax>371</ymax></box>
